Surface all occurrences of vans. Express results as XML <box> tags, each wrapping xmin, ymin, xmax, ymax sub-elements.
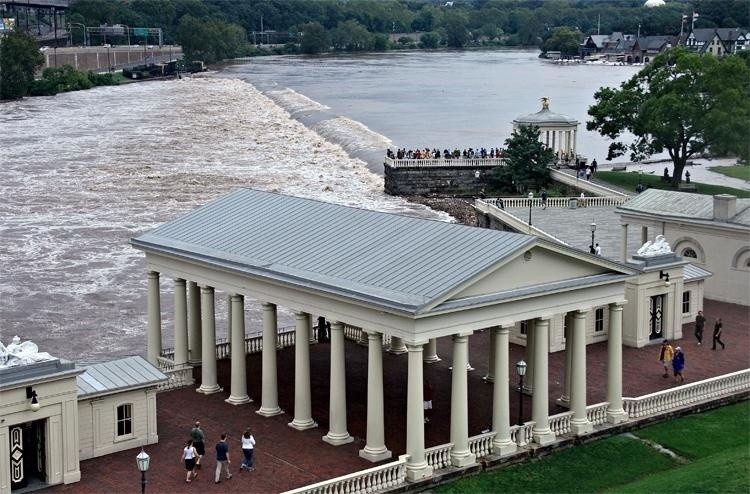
<box><xmin>103</xmin><ymin>43</ymin><xmax>112</xmax><ymax>48</ymax></box>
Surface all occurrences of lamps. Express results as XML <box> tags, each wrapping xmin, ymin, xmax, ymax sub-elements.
<box><xmin>658</xmin><ymin>270</ymin><xmax>671</xmax><ymax>289</ymax></box>
<box><xmin>23</xmin><ymin>384</ymin><xmax>39</xmax><ymax>413</ymax></box>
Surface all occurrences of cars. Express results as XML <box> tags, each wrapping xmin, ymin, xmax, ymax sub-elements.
<box><xmin>39</xmin><ymin>46</ymin><xmax>51</xmax><ymax>54</ymax></box>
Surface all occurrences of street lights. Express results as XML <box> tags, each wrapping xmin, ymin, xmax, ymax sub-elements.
<box><xmin>588</xmin><ymin>220</ymin><xmax>598</xmax><ymax>253</ymax></box>
<box><xmin>516</xmin><ymin>357</ymin><xmax>528</xmax><ymax>427</ymax></box>
<box><xmin>526</xmin><ymin>192</ymin><xmax>535</xmax><ymax>226</ymax></box>
<box><xmin>135</xmin><ymin>445</ymin><xmax>152</xmax><ymax>493</ymax></box>
<box><xmin>475</xmin><ymin>169</ymin><xmax>480</xmax><ymax>199</ymax></box>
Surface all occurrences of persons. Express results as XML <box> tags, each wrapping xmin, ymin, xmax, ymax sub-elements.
<box><xmin>664</xmin><ymin>167</ymin><xmax>668</xmax><ymax>179</ymax></box>
<box><xmin>423</xmin><ymin>362</ymin><xmax>434</xmax><ymax>424</ymax></box>
<box><xmin>658</xmin><ymin>339</ymin><xmax>674</xmax><ymax>378</ymax></box>
<box><xmin>710</xmin><ymin>317</ymin><xmax>725</xmax><ymax>350</ymax></box>
<box><xmin>589</xmin><ymin>245</ymin><xmax>595</xmax><ymax>254</ymax></box>
<box><xmin>673</xmin><ymin>346</ymin><xmax>684</xmax><ymax>383</ymax></box>
<box><xmin>685</xmin><ymin>170</ymin><xmax>690</xmax><ymax>184</ymax></box>
<box><xmin>387</xmin><ymin>148</ymin><xmax>598</xmax><ymax>181</ymax></box>
<box><xmin>180</xmin><ymin>421</ymin><xmax>256</xmax><ymax>482</ymax></box>
<box><xmin>581</xmin><ymin>191</ymin><xmax>584</xmax><ymax>197</ymax></box>
<box><xmin>479</xmin><ymin>188</ymin><xmax>487</xmax><ymax>199</ymax></box>
<box><xmin>593</xmin><ymin>243</ymin><xmax>601</xmax><ymax>257</ymax></box>
<box><xmin>496</xmin><ymin>198</ymin><xmax>500</xmax><ymax>208</ymax></box>
<box><xmin>500</xmin><ymin>199</ymin><xmax>504</xmax><ymax>210</ymax></box>
<box><xmin>638</xmin><ymin>182</ymin><xmax>642</xmax><ymax>193</ymax></box>
<box><xmin>694</xmin><ymin>311</ymin><xmax>707</xmax><ymax>345</ymax></box>
<box><xmin>542</xmin><ymin>191</ymin><xmax>547</xmax><ymax>210</ymax></box>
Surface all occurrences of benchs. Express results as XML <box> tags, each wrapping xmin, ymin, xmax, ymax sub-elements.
<box><xmin>611</xmin><ymin>166</ymin><xmax>627</xmax><ymax>172</ymax></box>
<box><xmin>678</xmin><ymin>182</ymin><xmax>698</xmax><ymax>193</ymax></box>
